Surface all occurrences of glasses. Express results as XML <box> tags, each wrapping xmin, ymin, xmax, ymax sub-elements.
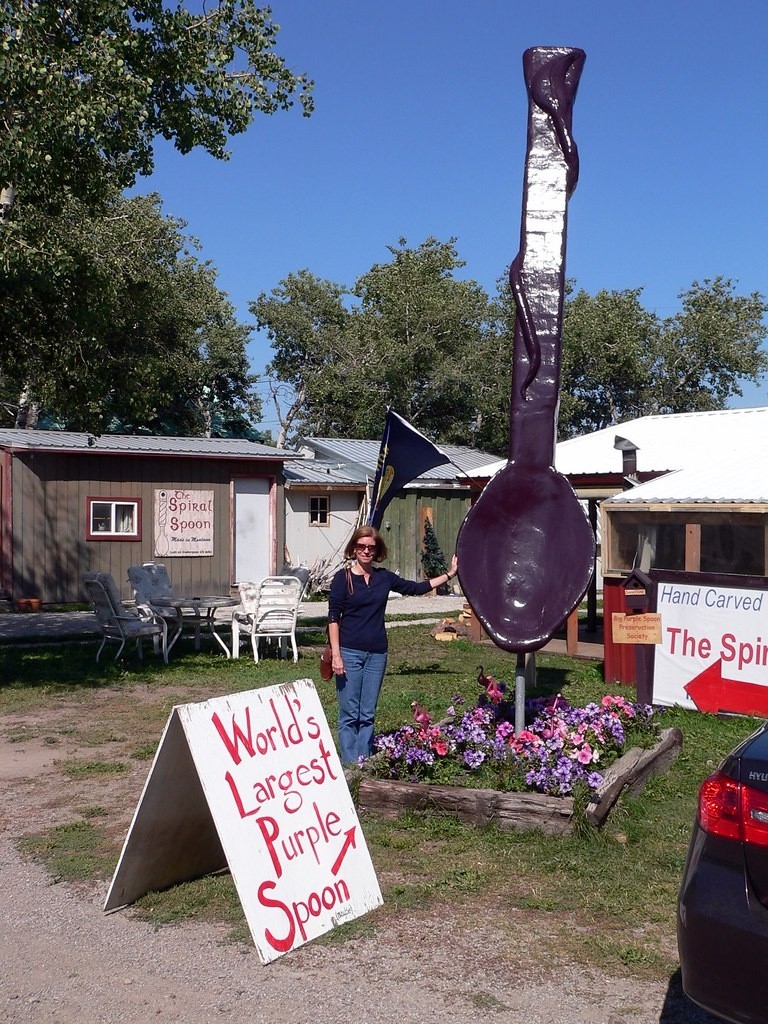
<box><xmin>355</xmin><ymin>543</ymin><xmax>376</xmax><ymax>554</ymax></box>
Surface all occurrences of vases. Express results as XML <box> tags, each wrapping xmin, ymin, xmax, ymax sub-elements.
<box><xmin>15</xmin><ymin>599</ymin><xmax>43</xmax><ymax>612</ymax></box>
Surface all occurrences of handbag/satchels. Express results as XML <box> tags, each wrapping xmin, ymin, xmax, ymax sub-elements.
<box><xmin>320</xmin><ymin>645</ymin><xmax>334</xmax><ymax>682</ymax></box>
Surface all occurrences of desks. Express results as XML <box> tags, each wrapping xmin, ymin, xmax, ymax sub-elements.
<box><xmin>148</xmin><ymin>595</ymin><xmax>242</xmax><ymax>663</ymax></box>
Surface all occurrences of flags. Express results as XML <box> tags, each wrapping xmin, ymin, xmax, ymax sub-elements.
<box><xmin>367</xmin><ymin>412</ymin><xmax>455</xmax><ymax>531</ymax></box>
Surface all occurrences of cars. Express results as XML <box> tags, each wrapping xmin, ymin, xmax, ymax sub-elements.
<box><xmin>676</xmin><ymin>722</ymin><xmax>767</xmax><ymax>1024</ymax></box>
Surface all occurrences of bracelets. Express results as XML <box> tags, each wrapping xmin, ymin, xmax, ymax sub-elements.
<box><xmin>445</xmin><ymin>573</ymin><xmax>452</xmax><ymax>580</ymax></box>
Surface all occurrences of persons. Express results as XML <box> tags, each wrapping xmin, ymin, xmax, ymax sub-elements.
<box><xmin>328</xmin><ymin>526</ymin><xmax>458</xmax><ymax>767</ymax></box>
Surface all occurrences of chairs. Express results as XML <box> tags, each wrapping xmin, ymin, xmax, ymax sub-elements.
<box><xmin>80</xmin><ymin>571</ymin><xmax>167</xmax><ymax>664</ymax></box>
<box><xmin>240</xmin><ymin>566</ymin><xmax>310</xmax><ymax>648</ymax></box>
<box><xmin>232</xmin><ymin>575</ymin><xmax>302</xmax><ymax>665</ymax></box>
<box><xmin>126</xmin><ymin>563</ymin><xmax>181</xmax><ymax>649</ymax></box>
